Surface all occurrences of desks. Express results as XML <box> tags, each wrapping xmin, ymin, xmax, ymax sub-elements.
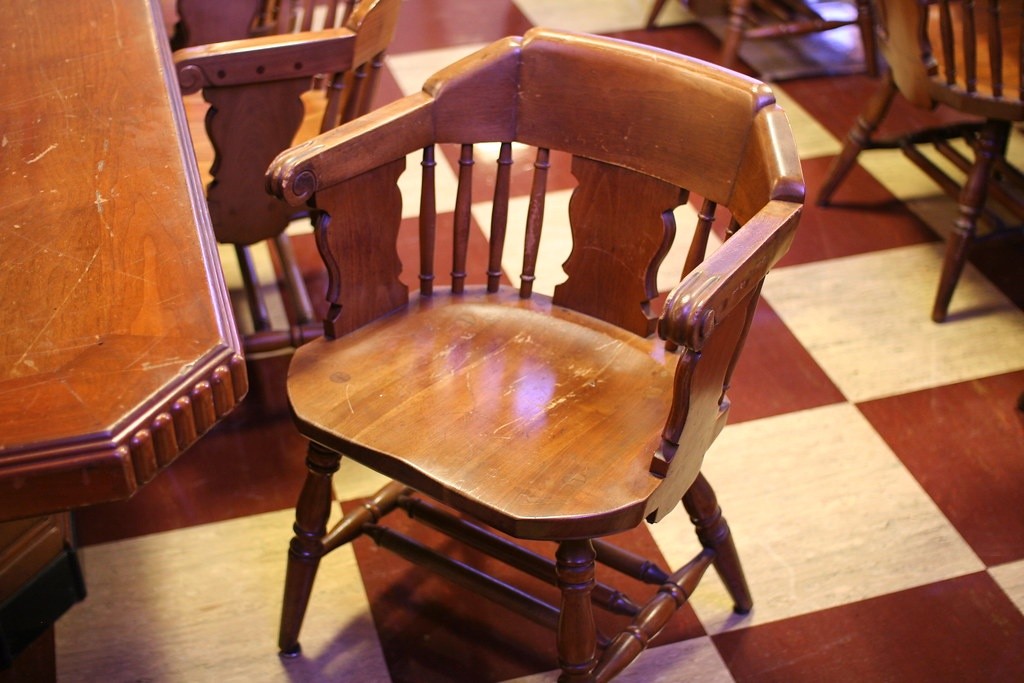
<box><xmin>0</xmin><ymin>0</ymin><xmax>250</xmax><ymax>683</ymax></box>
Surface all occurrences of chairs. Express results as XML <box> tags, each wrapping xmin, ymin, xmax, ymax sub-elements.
<box><xmin>813</xmin><ymin>0</ymin><xmax>1024</xmax><ymax>322</ymax></box>
<box><xmin>262</xmin><ymin>28</ymin><xmax>805</xmax><ymax>683</ymax></box>
<box><xmin>648</xmin><ymin>0</ymin><xmax>879</xmax><ymax>78</ymax></box>
<box><xmin>166</xmin><ymin>0</ymin><xmax>400</xmax><ymax>424</ymax></box>
